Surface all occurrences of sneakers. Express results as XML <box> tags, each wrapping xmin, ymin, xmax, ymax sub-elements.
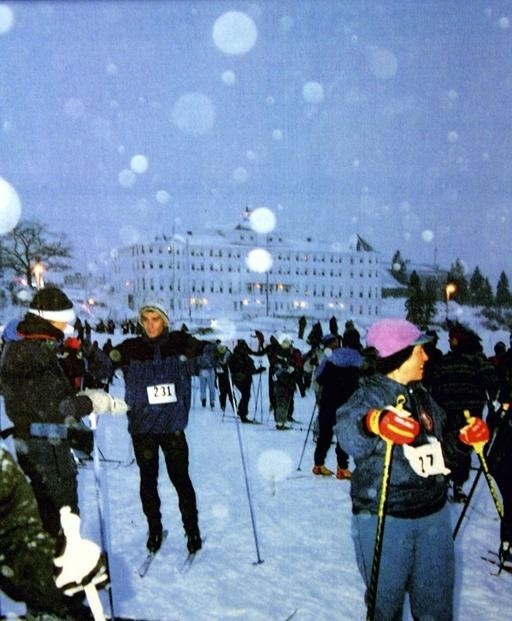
<box><xmin>186</xmin><ymin>528</ymin><xmax>202</xmax><ymax>553</ymax></box>
<box><xmin>452</xmin><ymin>486</ymin><xmax>468</xmax><ymax>503</ymax></box>
<box><xmin>146</xmin><ymin>525</ymin><xmax>163</xmax><ymax>552</ymax></box>
<box><xmin>336</xmin><ymin>466</ymin><xmax>352</xmax><ymax>479</ymax></box>
<box><xmin>312</xmin><ymin>466</ymin><xmax>334</xmax><ymax>476</ymax></box>
<box><xmin>498</xmin><ymin>542</ymin><xmax>511</xmax><ymax>569</ymax></box>
<box><xmin>276</xmin><ymin>424</ymin><xmax>290</xmax><ymax>430</ymax></box>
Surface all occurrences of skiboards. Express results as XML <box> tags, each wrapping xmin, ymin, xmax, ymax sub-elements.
<box><xmin>256</xmin><ymin>419</ymin><xmax>314</xmax><ymax>431</ymax></box>
<box><xmin>76</xmin><ymin>457</ymin><xmax>135</xmax><ymax>471</ymax></box>
<box><xmin>481</xmin><ymin>550</ymin><xmax>512</xmax><ymax>574</ymax></box>
<box><xmin>223</xmin><ymin>414</ymin><xmax>261</xmax><ymax>424</ymax></box>
<box><xmin>137</xmin><ymin>529</ymin><xmax>202</xmax><ymax>578</ymax></box>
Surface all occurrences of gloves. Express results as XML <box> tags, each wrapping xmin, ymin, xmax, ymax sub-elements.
<box><xmin>102</xmin><ymin>338</ymin><xmax>115</xmax><ymax>359</ymax></box>
<box><xmin>89</xmin><ymin>393</ymin><xmax>112</xmax><ymax>416</ymax></box>
<box><xmin>216</xmin><ymin>345</ymin><xmax>234</xmax><ymax>366</ymax></box>
<box><xmin>365</xmin><ymin>404</ymin><xmax>420</xmax><ymax>446</ymax></box>
<box><xmin>458</xmin><ymin>418</ymin><xmax>491</xmax><ymax>448</ymax></box>
<box><xmin>51</xmin><ymin>528</ymin><xmax>111</xmax><ymax>600</ymax></box>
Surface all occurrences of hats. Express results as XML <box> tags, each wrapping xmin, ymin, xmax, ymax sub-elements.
<box><xmin>365</xmin><ymin>317</ymin><xmax>434</xmax><ymax>361</ymax></box>
<box><xmin>321</xmin><ymin>334</ymin><xmax>337</xmax><ymax>346</ymax></box>
<box><xmin>138</xmin><ymin>303</ymin><xmax>170</xmax><ymax>330</ymax></box>
<box><xmin>456</xmin><ymin>331</ymin><xmax>480</xmax><ymax>351</ymax></box>
<box><xmin>28</xmin><ymin>287</ymin><xmax>76</xmax><ymax>324</ymax></box>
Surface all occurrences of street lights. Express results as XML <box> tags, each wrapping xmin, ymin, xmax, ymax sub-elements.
<box><xmin>252</xmin><ymin>282</ymin><xmax>284</xmax><ymax>316</ymax></box>
<box><xmin>32</xmin><ymin>262</ymin><xmax>45</xmax><ymax>293</ymax></box>
<box><xmin>445</xmin><ymin>284</ymin><xmax>456</xmax><ymax>320</ymax></box>
